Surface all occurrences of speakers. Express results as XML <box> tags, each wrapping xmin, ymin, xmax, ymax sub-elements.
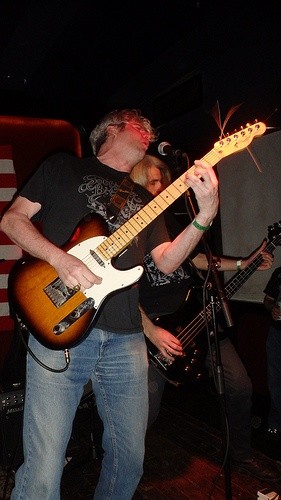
<box><xmin>0</xmin><ymin>379</ymin><xmax>24</xmax><ymax>471</ymax></box>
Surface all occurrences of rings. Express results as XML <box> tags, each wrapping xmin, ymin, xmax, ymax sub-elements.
<box><xmin>73</xmin><ymin>283</ymin><xmax>81</xmax><ymax>292</ymax></box>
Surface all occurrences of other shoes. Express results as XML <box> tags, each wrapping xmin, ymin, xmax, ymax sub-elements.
<box><xmin>230</xmin><ymin>448</ymin><xmax>281</xmax><ymax>484</ymax></box>
<box><xmin>264</xmin><ymin>424</ymin><xmax>281</xmax><ymax>441</ymax></box>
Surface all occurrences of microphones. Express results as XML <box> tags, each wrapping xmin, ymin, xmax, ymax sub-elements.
<box><xmin>158</xmin><ymin>142</ymin><xmax>186</xmax><ymax>157</ymax></box>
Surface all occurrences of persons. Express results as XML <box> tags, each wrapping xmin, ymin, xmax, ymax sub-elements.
<box><xmin>0</xmin><ymin>108</ymin><xmax>220</xmax><ymax>500</ymax></box>
<box><xmin>262</xmin><ymin>266</ymin><xmax>281</xmax><ymax>437</ymax></box>
<box><xmin>104</xmin><ymin>150</ymin><xmax>260</xmax><ymax>464</ymax></box>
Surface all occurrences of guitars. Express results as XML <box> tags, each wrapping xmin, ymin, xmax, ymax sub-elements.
<box><xmin>6</xmin><ymin>118</ymin><xmax>270</xmax><ymax>353</ymax></box>
<box><xmin>144</xmin><ymin>219</ymin><xmax>281</xmax><ymax>385</ymax></box>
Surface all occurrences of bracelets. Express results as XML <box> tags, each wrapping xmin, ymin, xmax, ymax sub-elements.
<box><xmin>190</xmin><ymin>218</ymin><xmax>214</xmax><ymax>231</ymax></box>
<box><xmin>236</xmin><ymin>259</ymin><xmax>243</xmax><ymax>272</ymax></box>
